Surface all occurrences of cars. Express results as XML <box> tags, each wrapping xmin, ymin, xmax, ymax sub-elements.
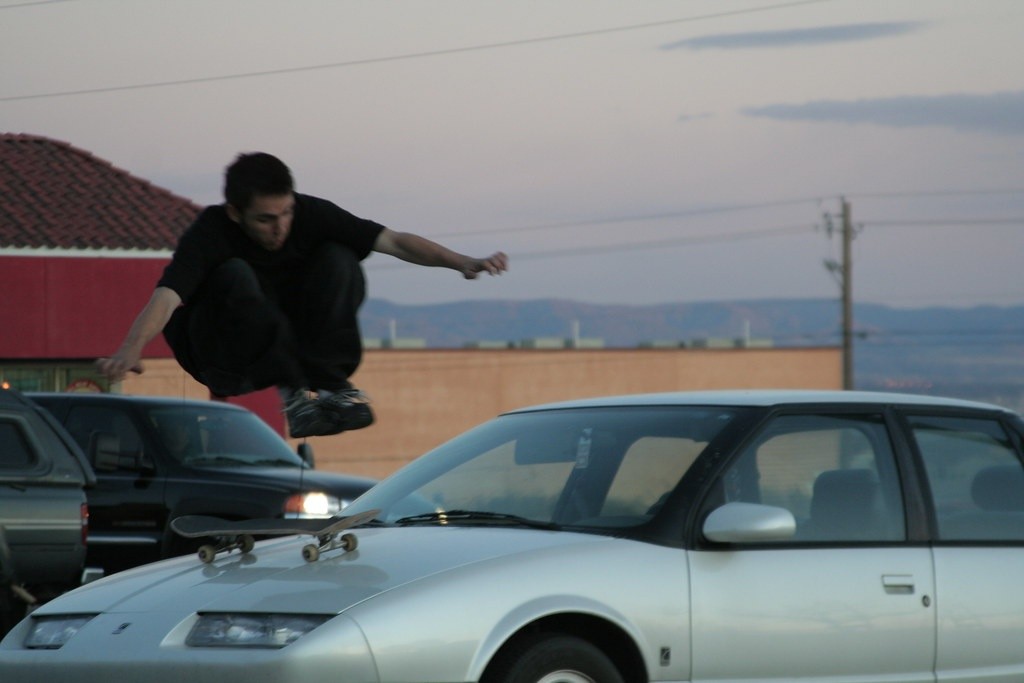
<box><xmin>1</xmin><ymin>389</ymin><xmax>1024</xmax><ymax>683</ymax></box>
<box><xmin>0</xmin><ymin>385</ymin><xmax>98</xmax><ymax>635</ymax></box>
<box><xmin>23</xmin><ymin>391</ymin><xmax>432</xmax><ymax>585</ymax></box>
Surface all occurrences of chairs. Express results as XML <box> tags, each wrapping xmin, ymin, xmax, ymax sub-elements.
<box><xmin>960</xmin><ymin>463</ymin><xmax>1024</xmax><ymax>541</ymax></box>
<box><xmin>802</xmin><ymin>468</ymin><xmax>892</xmax><ymax>541</ymax></box>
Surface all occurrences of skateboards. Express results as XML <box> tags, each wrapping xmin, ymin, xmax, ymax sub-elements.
<box><xmin>170</xmin><ymin>509</ymin><xmax>380</xmax><ymax>562</ymax></box>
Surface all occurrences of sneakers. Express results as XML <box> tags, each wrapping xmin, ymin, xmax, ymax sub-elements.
<box><xmin>281</xmin><ymin>381</ymin><xmax>373</xmax><ymax>438</ymax></box>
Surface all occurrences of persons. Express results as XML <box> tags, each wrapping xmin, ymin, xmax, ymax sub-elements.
<box><xmin>95</xmin><ymin>152</ymin><xmax>510</xmax><ymax>440</ymax></box>
